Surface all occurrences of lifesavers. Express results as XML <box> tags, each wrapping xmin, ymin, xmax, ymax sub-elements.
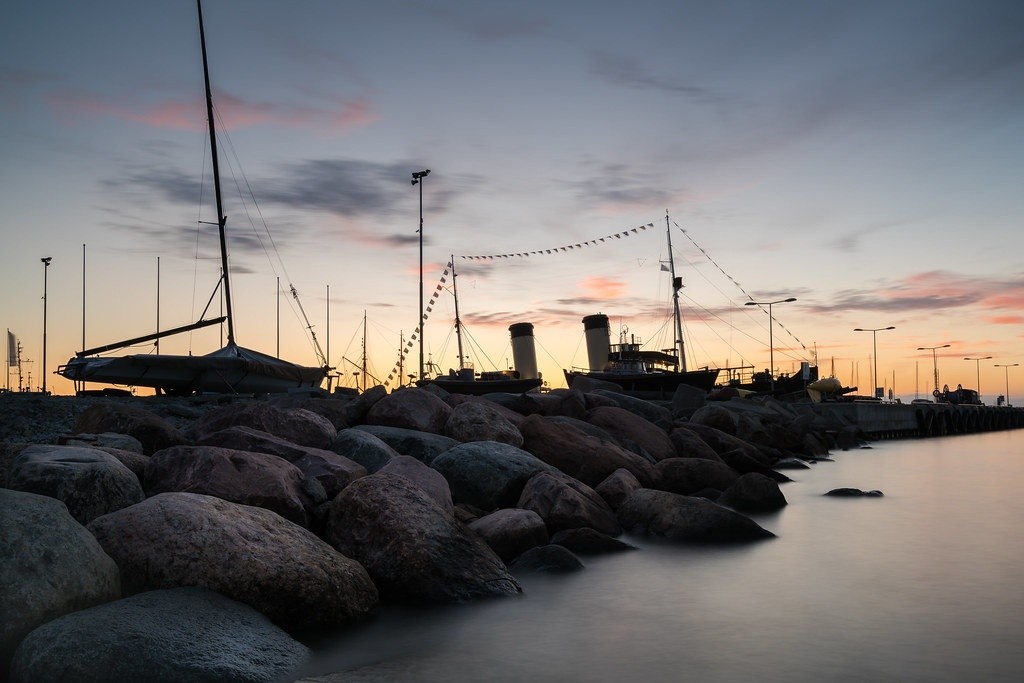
<box><xmin>103</xmin><ymin>388</ymin><xmax>132</xmax><ymax>396</ymax></box>
<box><xmin>544</xmin><ymin>381</ymin><xmax>547</xmax><ymax>386</ymax></box>
<box><xmin>77</xmin><ymin>390</ymin><xmax>104</xmax><ymax>396</ymax></box>
<box><xmin>933</xmin><ymin>390</ymin><xmax>940</xmax><ymax>397</ymax></box>
<box><xmin>513</xmin><ymin>371</ymin><xmax>520</xmax><ymax>379</ymax></box>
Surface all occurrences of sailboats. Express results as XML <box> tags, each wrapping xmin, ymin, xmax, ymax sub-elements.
<box><xmin>52</xmin><ymin>0</ymin><xmax>338</xmax><ymax>395</ymax></box>
<box><xmin>562</xmin><ymin>208</ymin><xmax>721</xmax><ymax>395</ymax></box>
<box><xmin>414</xmin><ymin>252</ymin><xmax>543</xmax><ymax>397</ymax></box>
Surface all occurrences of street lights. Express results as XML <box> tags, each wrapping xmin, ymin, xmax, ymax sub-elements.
<box><xmin>963</xmin><ymin>357</ymin><xmax>993</xmax><ymax>401</ymax></box>
<box><xmin>994</xmin><ymin>363</ymin><xmax>1020</xmax><ymax>405</ymax></box>
<box><xmin>853</xmin><ymin>326</ymin><xmax>896</xmax><ymax>400</ymax></box>
<box><xmin>917</xmin><ymin>344</ymin><xmax>951</xmax><ymax>403</ymax></box>
<box><xmin>41</xmin><ymin>256</ymin><xmax>52</xmax><ymax>395</ymax></box>
<box><xmin>411</xmin><ymin>169</ymin><xmax>432</xmax><ymax>380</ymax></box>
<box><xmin>744</xmin><ymin>297</ymin><xmax>797</xmax><ymax>398</ymax></box>
